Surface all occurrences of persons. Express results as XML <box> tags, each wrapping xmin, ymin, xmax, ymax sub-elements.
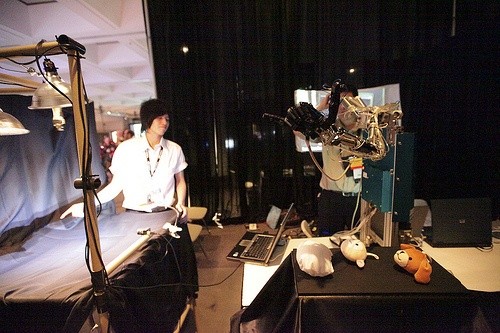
<box><xmin>292</xmin><ymin>83</ymin><xmax>363</xmax><ymax>238</ymax></box>
<box><xmin>60</xmin><ymin>99</ymin><xmax>188</xmax><ymax>219</ymax></box>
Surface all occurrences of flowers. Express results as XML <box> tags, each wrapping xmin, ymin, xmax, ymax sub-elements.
<box><xmin>98</xmin><ymin>136</ymin><xmax>118</xmax><ymax>160</ymax></box>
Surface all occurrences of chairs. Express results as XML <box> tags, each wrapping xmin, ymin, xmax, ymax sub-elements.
<box><xmin>186</xmin><ymin>206</ymin><xmax>210</xmax><ymax>234</ymax></box>
<box><xmin>187</xmin><ymin>223</ymin><xmax>208</xmax><ymax>259</ymax></box>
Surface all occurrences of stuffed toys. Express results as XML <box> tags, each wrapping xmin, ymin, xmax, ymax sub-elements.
<box><xmin>340</xmin><ymin>238</ymin><xmax>367</xmax><ymax>267</ymax></box>
<box><xmin>296</xmin><ymin>240</ymin><xmax>334</xmax><ymax>277</ymax></box>
<box><xmin>394</xmin><ymin>244</ymin><xmax>432</xmax><ymax>283</ymax></box>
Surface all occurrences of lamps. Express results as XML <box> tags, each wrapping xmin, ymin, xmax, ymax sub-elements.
<box><xmin>28</xmin><ymin>56</ymin><xmax>93</xmax><ymax>132</ymax></box>
<box><xmin>0</xmin><ymin>108</ymin><xmax>30</xmax><ymax>136</ymax></box>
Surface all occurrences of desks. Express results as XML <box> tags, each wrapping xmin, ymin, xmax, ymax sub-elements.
<box><xmin>0</xmin><ymin>208</ymin><xmax>199</xmax><ymax>333</ymax></box>
<box><xmin>241</xmin><ymin>236</ymin><xmax>341</xmax><ymax>306</ymax></box>
<box><xmin>230</xmin><ymin>232</ymin><xmax>500</xmax><ymax>333</ymax></box>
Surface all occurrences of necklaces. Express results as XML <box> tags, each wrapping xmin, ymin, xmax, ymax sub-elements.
<box><xmin>145</xmin><ymin>146</ymin><xmax>163</xmax><ymax>177</ymax></box>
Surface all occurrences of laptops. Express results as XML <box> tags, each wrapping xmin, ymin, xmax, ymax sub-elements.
<box><xmin>424</xmin><ymin>198</ymin><xmax>493</xmax><ymax>247</ymax></box>
<box><xmin>226</xmin><ymin>202</ymin><xmax>294</xmax><ymax>267</ymax></box>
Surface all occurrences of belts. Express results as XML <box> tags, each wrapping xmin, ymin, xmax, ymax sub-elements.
<box><xmin>323</xmin><ymin>189</ymin><xmax>358</xmax><ymax>196</ymax></box>
